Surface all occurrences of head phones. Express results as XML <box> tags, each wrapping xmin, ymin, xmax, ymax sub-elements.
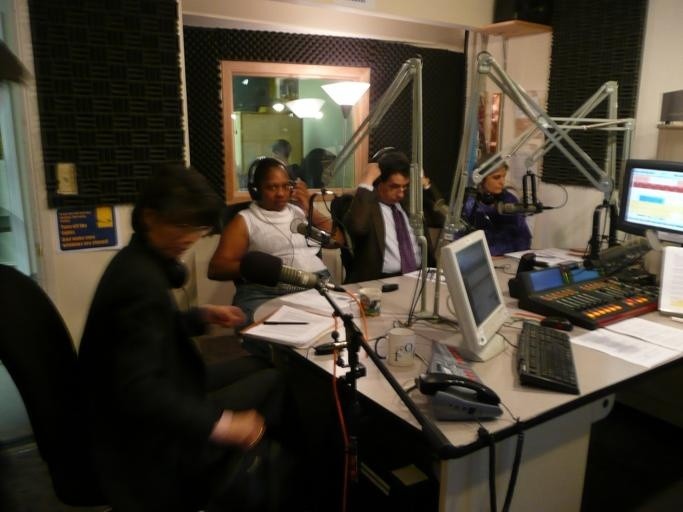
<box><xmin>372</xmin><ymin>147</ymin><xmax>404</xmax><ymax>185</ymax></box>
<box><xmin>133</xmin><ymin>233</ymin><xmax>190</xmax><ymax>289</ymax></box>
<box><xmin>248</xmin><ymin>158</ymin><xmax>294</xmax><ymax>200</ymax></box>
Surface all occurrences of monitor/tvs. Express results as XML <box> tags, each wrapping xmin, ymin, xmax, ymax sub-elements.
<box><xmin>439</xmin><ymin>229</ymin><xmax>509</xmax><ymax>362</ymax></box>
<box><xmin>616</xmin><ymin>159</ymin><xmax>683</xmax><ymax>245</ymax></box>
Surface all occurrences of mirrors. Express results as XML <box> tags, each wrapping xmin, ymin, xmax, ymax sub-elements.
<box><xmin>221</xmin><ymin>61</ymin><xmax>369</xmax><ymax>208</ymax></box>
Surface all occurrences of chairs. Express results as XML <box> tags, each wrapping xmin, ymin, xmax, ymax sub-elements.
<box><xmin>215</xmin><ymin>200</ymin><xmax>249</xmax><ymax>231</ymax></box>
<box><xmin>1</xmin><ymin>258</ymin><xmax>111</xmax><ymax>512</ymax></box>
<box><xmin>329</xmin><ymin>195</ymin><xmax>356</xmax><ymax>285</ymax></box>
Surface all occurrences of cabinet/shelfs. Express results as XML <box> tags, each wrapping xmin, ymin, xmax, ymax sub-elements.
<box><xmin>233</xmin><ymin>112</ymin><xmax>304</xmax><ymax>174</ymax></box>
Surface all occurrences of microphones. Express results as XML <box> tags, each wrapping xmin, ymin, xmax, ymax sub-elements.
<box><xmin>431</xmin><ymin>195</ymin><xmax>467</xmax><ymax>231</ymax></box>
<box><xmin>497</xmin><ymin>201</ymin><xmax>542</xmax><ymax>214</ymax></box>
<box><xmin>240</xmin><ymin>251</ymin><xmax>317</xmax><ymax>290</ymax></box>
<box><xmin>290</xmin><ymin>218</ymin><xmax>338</xmax><ymax>249</ymax></box>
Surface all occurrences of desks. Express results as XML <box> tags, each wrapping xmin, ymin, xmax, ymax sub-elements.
<box><xmin>250</xmin><ymin>249</ymin><xmax>683</xmax><ymax>512</ymax></box>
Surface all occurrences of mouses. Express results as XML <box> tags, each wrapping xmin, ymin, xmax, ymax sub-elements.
<box><xmin>541</xmin><ymin>315</ymin><xmax>572</xmax><ymax>331</ymax></box>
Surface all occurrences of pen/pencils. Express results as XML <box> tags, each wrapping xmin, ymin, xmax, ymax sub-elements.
<box><xmin>263</xmin><ymin>321</ymin><xmax>311</xmax><ymax>324</ymax></box>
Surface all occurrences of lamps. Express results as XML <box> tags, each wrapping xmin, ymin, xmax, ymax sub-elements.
<box><xmin>320</xmin><ymin>82</ymin><xmax>371</xmax><ymax>284</ymax></box>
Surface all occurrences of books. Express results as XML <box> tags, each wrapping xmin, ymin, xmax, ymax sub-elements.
<box><xmin>237</xmin><ymin>304</ymin><xmax>342</xmax><ymax>351</ymax></box>
<box><xmin>277</xmin><ymin>287</ymin><xmax>364</xmax><ymax>319</ymax></box>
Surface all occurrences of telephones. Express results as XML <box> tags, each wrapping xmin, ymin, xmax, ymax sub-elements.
<box><xmin>415</xmin><ymin>373</ymin><xmax>503</xmax><ymax>420</ymax></box>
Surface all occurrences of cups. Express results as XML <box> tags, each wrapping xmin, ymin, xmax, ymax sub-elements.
<box><xmin>348</xmin><ymin>286</ymin><xmax>382</xmax><ymax>317</ymax></box>
<box><xmin>374</xmin><ymin>328</ymin><xmax>416</xmax><ymax>367</ymax></box>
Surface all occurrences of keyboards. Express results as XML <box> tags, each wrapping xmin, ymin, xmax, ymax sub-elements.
<box><xmin>519</xmin><ymin>322</ymin><xmax>577</xmax><ymax>394</ymax></box>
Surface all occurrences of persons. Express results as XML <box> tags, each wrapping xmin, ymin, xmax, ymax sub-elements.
<box><xmin>344</xmin><ymin>150</ymin><xmax>450</xmax><ymax>284</ymax></box>
<box><xmin>299</xmin><ymin>147</ymin><xmax>336</xmax><ymax>187</ymax></box>
<box><xmin>76</xmin><ymin>163</ymin><xmax>281</xmax><ymax>512</ymax></box>
<box><xmin>453</xmin><ymin>153</ymin><xmax>533</xmax><ymax>258</ymax></box>
<box><xmin>268</xmin><ymin>139</ymin><xmax>292</xmax><ymax>163</ymax></box>
<box><xmin>205</xmin><ymin>155</ymin><xmax>345</xmax><ymax>369</ymax></box>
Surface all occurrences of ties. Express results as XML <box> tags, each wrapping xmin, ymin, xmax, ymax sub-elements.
<box><xmin>390</xmin><ymin>204</ymin><xmax>417</xmax><ymax>274</ymax></box>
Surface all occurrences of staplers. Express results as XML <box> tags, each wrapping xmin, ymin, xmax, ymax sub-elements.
<box><xmin>382</xmin><ymin>285</ymin><xmax>399</xmax><ymax>292</ymax></box>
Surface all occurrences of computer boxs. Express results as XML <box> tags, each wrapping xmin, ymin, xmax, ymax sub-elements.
<box><xmin>357</xmin><ymin>464</ymin><xmax>439</xmax><ymax>512</ymax></box>
